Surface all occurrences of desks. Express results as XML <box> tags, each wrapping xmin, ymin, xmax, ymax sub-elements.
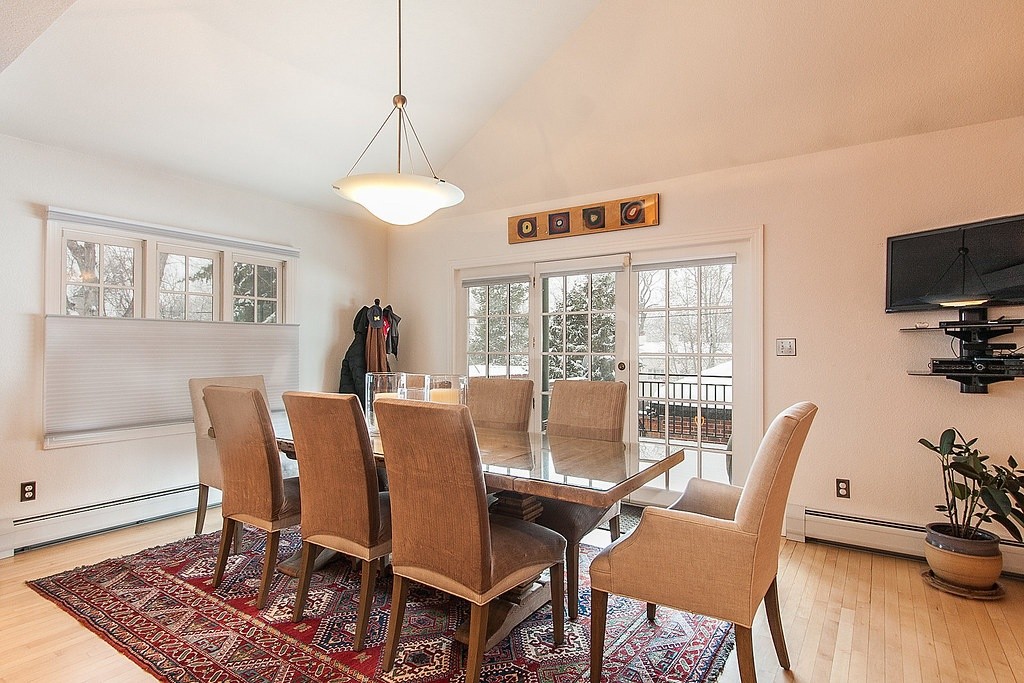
<box><xmin>208</xmin><ymin>416</ymin><xmax>685</xmax><ymax>653</ymax></box>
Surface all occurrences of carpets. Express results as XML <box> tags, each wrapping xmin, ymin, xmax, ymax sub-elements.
<box><xmin>25</xmin><ymin>529</ymin><xmax>738</xmax><ymax>683</ymax></box>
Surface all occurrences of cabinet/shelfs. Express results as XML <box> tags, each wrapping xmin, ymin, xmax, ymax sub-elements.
<box><xmin>900</xmin><ymin>323</ymin><xmax>1024</xmax><ymax>394</ymax></box>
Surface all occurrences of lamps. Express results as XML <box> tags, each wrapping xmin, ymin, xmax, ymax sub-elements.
<box><xmin>330</xmin><ymin>2</ymin><xmax>466</xmax><ymax>226</ymax></box>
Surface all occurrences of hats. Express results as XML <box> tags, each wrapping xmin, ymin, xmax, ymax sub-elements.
<box><xmin>367</xmin><ymin>305</ymin><xmax>382</xmax><ymax>328</ymax></box>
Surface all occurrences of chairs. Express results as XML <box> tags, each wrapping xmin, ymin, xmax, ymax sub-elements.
<box><xmin>374</xmin><ymin>397</ymin><xmax>567</xmax><ymax>683</ymax></box>
<box><xmin>282</xmin><ymin>390</ymin><xmax>392</xmax><ymax>653</ymax></box>
<box><xmin>589</xmin><ymin>401</ymin><xmax>818</xmax><ymax>682</ymax></box>
<box><xmin>464</xmin><ymin>377</ymin><xmax>534</xmax><ymax>494</ymax></box>
<box><xmin>189</xmin><ymin>375</ymin><xmax>271</xmax><ymax>553</ymax></box>
<box><xmin>202</xmin><ymin>384</ymin><xmax>302</xmax><ymax>609</ymax></box>
<box><xmin>488</xmin><ymin>381</ymin><xmax>627</xmax><ymax>617</ymax></box>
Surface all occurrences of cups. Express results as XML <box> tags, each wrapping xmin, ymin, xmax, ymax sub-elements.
<box><xmin>365</xmin><ymin>371</ymin><xmax>467</xmax><ymax>438</ymax></box>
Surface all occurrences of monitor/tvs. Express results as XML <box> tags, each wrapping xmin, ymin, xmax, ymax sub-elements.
<box><xmin>885</xmin><ymin>213</ymin><xmax>1024</xmax><ymax>314</ymax></box>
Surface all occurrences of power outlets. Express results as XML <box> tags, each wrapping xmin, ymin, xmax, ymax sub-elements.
<box><xmin>835</xmin><ymin>477</ymin><xmax>851</xmax><ymax>500</ymax></box>
<box><xmin>20</xmin><ymin>480</ymin><xmax>37</xmax><ymax>502</ymax></box>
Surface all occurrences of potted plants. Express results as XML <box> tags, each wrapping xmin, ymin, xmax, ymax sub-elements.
<box><xmin>917</xmin><ymin>427</ymin><xmax>1024</xmax><ymax>600</ymax></box>
